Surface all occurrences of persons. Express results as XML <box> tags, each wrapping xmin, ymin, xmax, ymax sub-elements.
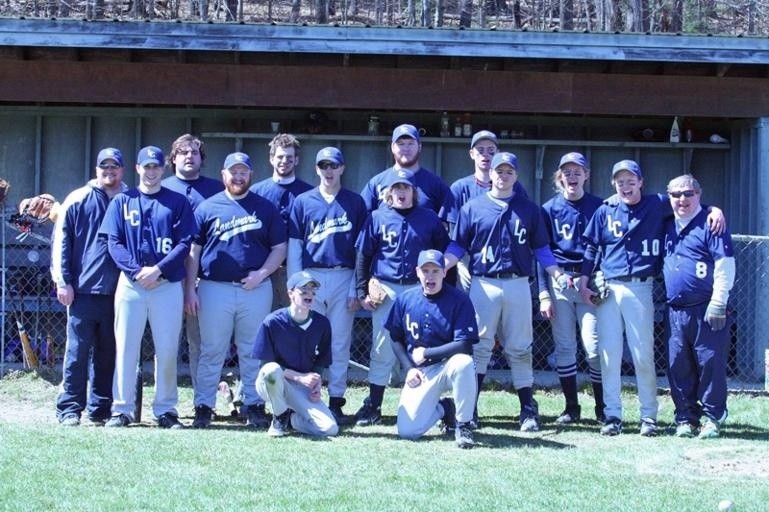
<box><xmin>251</xmin><ymin>271</ymin><xmax>338</xmax><ymax>436</ymax></box>
<box><xmin>359</xmin><ymin>125</ymin><xmax>735</xmax><ymax>440</ymax></box>
<box><xmin>384</xmin><ymin>250</ymin><xmax>480</xmax><ymax>449</ymax></box>
<box><xmin>49</xmin><ymin>124</ymin><xmax>367</xmax><ymax>428</ymax></box>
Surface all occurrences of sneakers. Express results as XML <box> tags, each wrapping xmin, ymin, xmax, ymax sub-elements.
<box><xmin>192</xmin><ymin>405</ymin><xmax>213</xmax><ymax>428</ymax></box>
<box><xmin>518</xmin><ymin>413</ymin><xmax>538</xmax><ymax>431</ymax></box>
<box><xmin>676</xmin><ymin>415</ymin><xmax>720</xmax><ymax>438</ymax></box>
<box><xmin>556</xmin><ymin>405</ymin><xmax>581</xmax><ymax>423</ymax></box>
<box><xmin>437</xmin><ymin>397</ymin><xmax>478</xmax><ymax>448</ymax></box>
<box><xmin>356</xmin><ymin>411</ymin><xmax>383</xmax><ymax>426</ymax></box>
<box><xmin>594</xmin><ymin>405</ymin><xmax>623</xmax><ymax>436</ymax></box>
<box><xmin>330</xmin><ymin>407</ymin><xmax>351</xmax><ymax>425</ymax></box>
<box><xmin>60</xmin><ymin>415</ymin><xmax>129</xmax><ymax>428</ymax></box>
<box><xmin>639</xmin><ymin>418</ymin><xmax>658</xmax><ymax>436</ymax></box>
<box><xmin>245</xmin><ymin>408</ymin><xmax>293</xmax><ymax>437</ymax></box>
<box><xmin>158</xmin><ymin>414</ymin><xmax>184</xmax><ymax>429</ymax></box>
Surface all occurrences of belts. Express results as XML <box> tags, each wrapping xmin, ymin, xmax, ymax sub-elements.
<box><xmin>671</xmin><ymin>303</ymin><xmax>702</xmax><ymax>309</ymax></box>
<box><xmin>615</xmin><ymin>277</ymin><xmax>646</xmax><ymax>283</ymax></box>
<box><xmin>395</xmin><ymin>279</ymin><xmax>418</xmax><ymax>285</ymax></box>
<box><xmin>227</xmin><ymin>278</ymin><xmax>246</xmax><ymax>284</ymax></box>
<box><xmin>483</xmin><ymin>273</ymin><xmax>519</xmax><ymax>278</ymax></box>
<box><xmin>564</xmin><ymin>265</ymin><xmax>582</xmax><ymax>272</ymax></box>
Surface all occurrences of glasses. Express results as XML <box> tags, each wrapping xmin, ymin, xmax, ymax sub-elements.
<box><xmin>669</xmin><ymin>190</ymin><xmax>695</xmax><ymax>198</ymax></box>
<box><xmin>318</xmin><ymin>161</ymin><xmax>339</xmax><ymax>170</ymax></box>
<box><xmin>298</xmin><ymin>286</ymin><xmax>317</xmax><ymax>292</ymax></box>
<box><xmin>98</xmin><ymin>164</ymin><xmax>122</xmax><ymax>169</ymax></box>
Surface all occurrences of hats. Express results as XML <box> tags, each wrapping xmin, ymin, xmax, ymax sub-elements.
<box><xmin>137</xmin><ymin>146</ymin><xmax>164</xmax><ymax>167</ymax></box>
<box><xmin>389</xmin><ymin>169</ymin><xmax>415</xmax><ymax>190</ymax></box>
<box><xmin>558</xmin><ymin>152</ymin><xmax>588</xmax><ymax>170</ymax></box>
<box><xmin>316</xmin><ymin>147</ymin><xmax>345</xmax><ymax>165</ymax></box>
<box><xmin>96</xmin><ymin>148</ymin><xmax>123</xmax><ymax>164</ymax></box>
<box><xmin>471</xmin><ymin>130</ymin><xmax>499</xmax><ymax>148</ymax></box>
<box><xmin>491</xmin><ymin>152</ymin><xmax>520</xmax><ymax>171</ymax></box>
<box><xmin>418</xmin><ymin>249</ymin><xmax>445</xmax><ymax>268</ymax></box>
<box><xmin>612</xmin><ymin>160</ymin><xmax>642</xmax><ymax>178</ymax></box>
<box><xmin>392</xmin><ymin>124</ymin><xmax>420</xmax><ymax>143</ymax></box>
<box><xmin>223</xmin><ymin>152</ymin><xmax>253</xmax><ymax>170</ymax></box>
<box><xmin>286</xmin><ymin>271</ymin><xmax>322</xmax><ymax>290</ymax></box>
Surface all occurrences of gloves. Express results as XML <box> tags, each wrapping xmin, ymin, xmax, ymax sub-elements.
<box><xmin>704</xmin><ymin>304</ymin><xmax>727</xmax><ymax>331</ymax></box>
